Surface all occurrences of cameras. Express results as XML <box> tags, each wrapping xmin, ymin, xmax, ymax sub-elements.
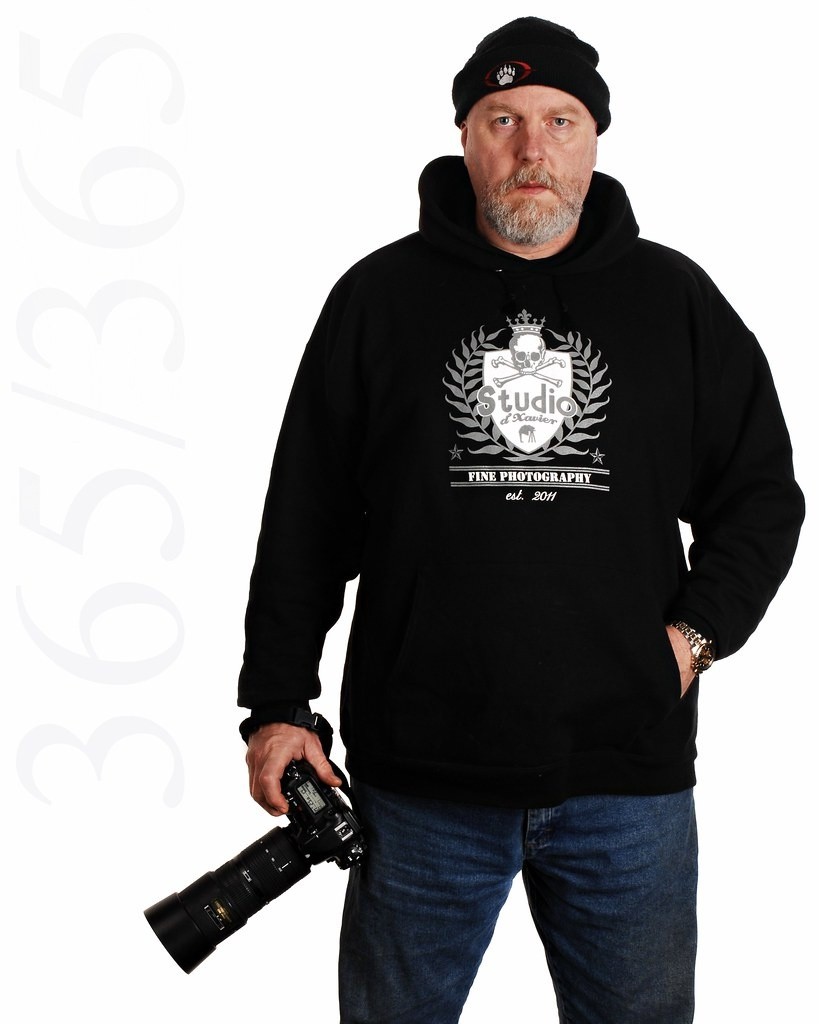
<box><xmin>142</xmin><ymin>712</ymin><xmax>369</xmax><ymax>975</ymax></box>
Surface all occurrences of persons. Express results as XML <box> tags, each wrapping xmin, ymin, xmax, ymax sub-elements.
<box><xmin>238</xmin><ymin>16</ymin><xmax>807</xmax><ymax>1024</ymax></box>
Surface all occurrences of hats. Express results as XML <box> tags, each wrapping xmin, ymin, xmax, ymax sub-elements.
<box><xmin>451</xmin><ymin>16</ymin><xmax>611</xmax><ymax>137</ymax></box>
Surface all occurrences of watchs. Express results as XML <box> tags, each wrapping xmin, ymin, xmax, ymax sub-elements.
<box><xmin>671</xmin><ymin>622</ymin><xmax>715</xmax><ymax>675</ymax></box>
